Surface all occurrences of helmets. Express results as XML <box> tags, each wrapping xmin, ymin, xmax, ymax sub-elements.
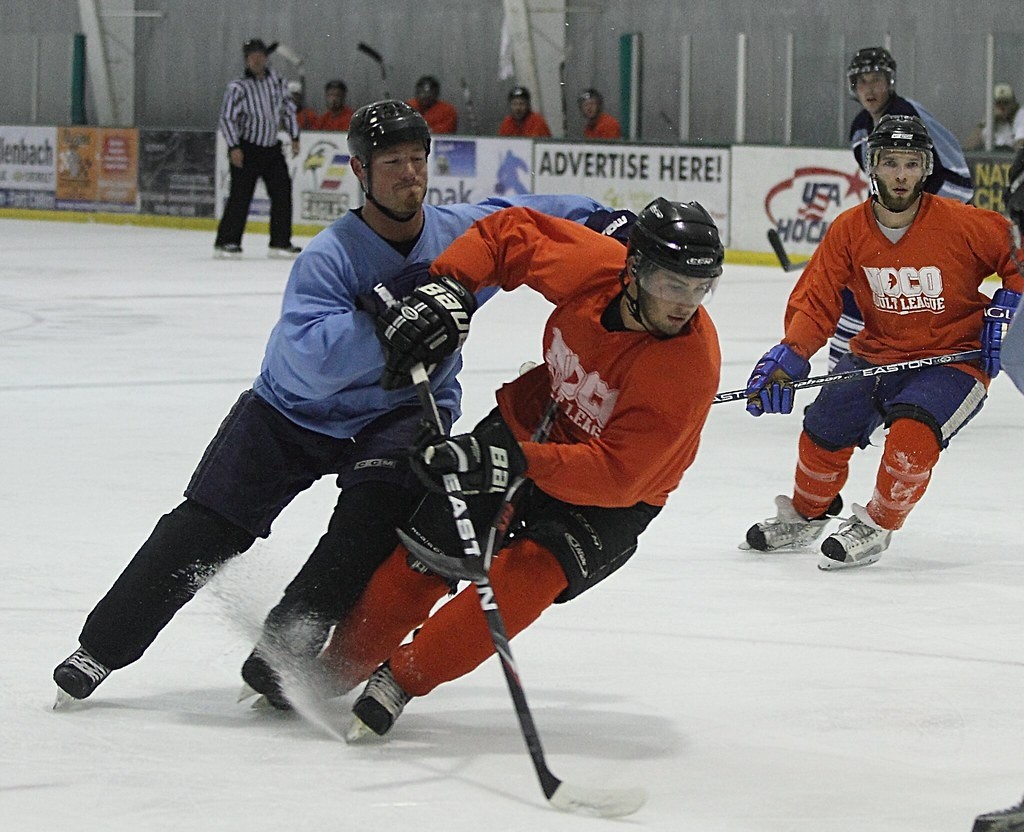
<box><xmin>347</xmin><ymin>100</ymin><xmax>431</xmax><ymax>164</ymax></box>
<box><xmin>846</xmin><ymin>48</ymin><xmax>897</xmax><ymax>100</ymax></box>
<box><xmin>630</xmin><ymin>197</ymin><xmax>725</xmax><ymax>305</ymax></box>
<box><xmin>866</xmin><ymin>113</ymin><xmax>934</xmax><ymax>183</ymax></box>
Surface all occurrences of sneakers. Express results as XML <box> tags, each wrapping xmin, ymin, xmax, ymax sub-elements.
<box><xmin>816</xmin><ymin>503</ymin><xmax>892</xmax><ymax>570</ymax></box>
<box><xmin>250</xmin><ymin>662</ymin><xmax>340</xmax><ymax>712</ymax></box>
<box><xmin>345</xmin><ymin>656</ymin><xmax>414</xmax><ymax>741</ymax></box>
<box><xmin>738</xmin><ymin>494</ymin><xmax>844</xmax><ymax>551</ymax></box>
<box><xmin>237</xmin><ymin>635</ymin><xmax>289</xmax><ymax>702</ymax></box>
<box><xmin>51</xmin><ymin>645</ymin><xmax>111</xmax><ymax>711</ymax></box>
<box><xmin>213</xmin><ymin>243</ymin><xmax>242</xmax><ymax>259</ymax></box>
<box><xmin>268</xmin><ymin>242</ymin><xmax>301</xmax><ymax>259</ymax></box>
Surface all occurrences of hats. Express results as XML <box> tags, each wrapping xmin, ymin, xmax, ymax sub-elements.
<box><xmin>416</xmin><ymin>75</ymin><xmax>439</xmax><ymax>91</ymax></box>
<box><xmin>508</xmin><ymin>86</ymin><xmax>531</xmax><ymax>101</ymax></box>
<box><xmin>992</xmin><ymin>83</ymin><xmax>1015</xmax><ymax>103</ymax></box>
<box><xmin>243</xmin><ymin>37</ymin><xmax>279</xmax><ymax>55</ymax></box>
<box><xmin>576</xmin><ymin>89</ymin><xmax>600</xmax><ymax>102</ymax></box>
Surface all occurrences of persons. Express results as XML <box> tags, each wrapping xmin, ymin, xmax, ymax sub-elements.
<box><xmin>746</xmin><ymin>115</ymin><xmax>1024</xmax><ymax>562</ymax></box>
<box><xmin>993</xmin><ymin>86</ymin><xmax>1024</xmax><ymax>149</ymax></box>
<box><xmin>213</xmin><ymin>38</ymin><xmax>301</xmax><ymax>255</ymax></box>
<box><xmin>496</xmin><ymin>86</ymin><xmax>553</xmax><ymax>136</ymax></box>
<box><xmin>576</xmin><ymin>87</ymin><xmax>623</xmax><ymax>140</ymax></box>
<box><xmin>51</xmin><ymin>100</ymin><xmax>725</xmax><ymax>736</ymax></box>
<box><xmin>279</xmin><ymin>79</ymin><xmax>316</xmax><ymax>128</ymax></box>
<box><xmin>308</xmin><ymin>81</ymin><xmax>357</xmax><ymax>132</ymax></box>
<box><xmin>846</xmin><ymin>49</ymin><xmax>976</xmax><ymax>204</ymax></box>
<box><xmin>405</xmin><ymin>76</ymin><xmax>457</xmax><ymax>134</ymax></box>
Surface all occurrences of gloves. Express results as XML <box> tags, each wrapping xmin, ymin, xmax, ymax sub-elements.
<box><xmin>410</xmin><ymin>424</ymin><xmax>528</xmax><ymax>497</ymax></box>
<box><xmin>355</xmin><ymin>262</ymin><xmax>433</xmax><ymax>317</ymax></box>
<box><xmin>978</xmin><ymin>288</ymin><xmax>1022</xmax><ymax>376</ymax></box>
<box><xmin>376</xmin><ymin>276</ymin><xmax>476</xmax><ymax>390</ymax></box>
<box><xmin>584</xmin><ymin>208</ymin><xmax>639</xmax><ymax>242</ymax></box>
<box><xmin>746</xmin><ymin>343</ymin><xmax>811</xmax><ymax>417</ymax></box>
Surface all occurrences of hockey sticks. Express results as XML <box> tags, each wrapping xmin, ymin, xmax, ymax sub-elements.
<box><xmin>460</xmin><ymin>75</ymin><xmax>481</xmax><ymax>136</ymax></box>
<box><xmin>766</xmin><ymin>228</ymin><xmax>810</xmax><ymax>274</ymax></box>
<box><xmin>272</xmin><ymin>40</ymin><xmax>307</xmax><ymax>109</ymax></box>
<box><xmin>356</xmin><ymin>40</ymin><xmax>391</xmax><ymax>99</ymax></box>
<box><xmin>516</xmin><ymin>348</ymin><xmax>987</xmax><ymax>405</ymax></box>
<box><xmin>558</xmin><ymin>53</ymin><xmax>577</xmax><ymax>138</ymax></box>
<box><xmin>407</xmin><ymin>361</ymin><xmax>651</xmax><ymax>821</ymax></box>
<box><xmin>658</xmin><ymin>110</ymin><xmax>680</xmax><ymax>144</ymax></box>
